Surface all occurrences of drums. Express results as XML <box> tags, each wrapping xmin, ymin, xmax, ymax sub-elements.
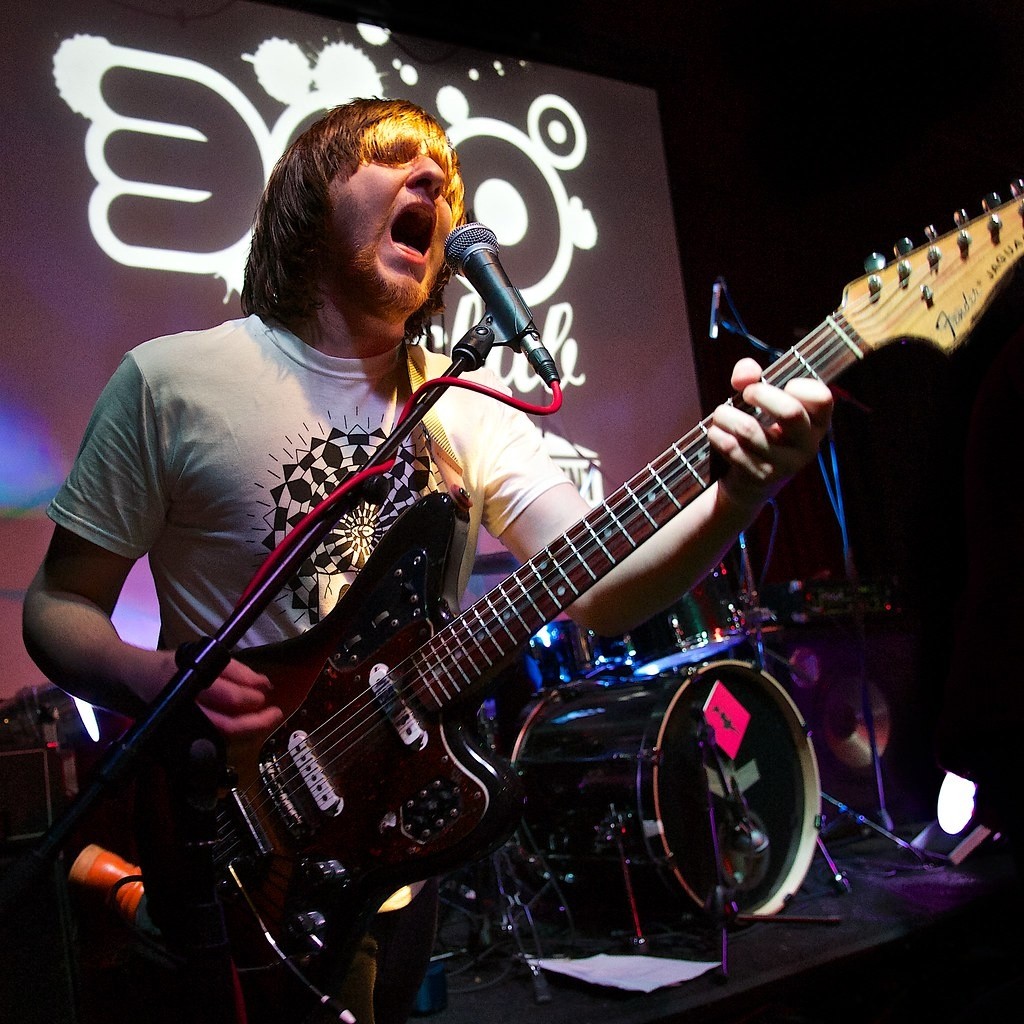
<box><xmin>517</xmin><ymin>608</ymin><xmax>640</xmax><ymax>701</ymax></box>
<box><xmin>0</xmin><ymin>681</ymin><xmax>132</xmax><ymax>849</ymax></box>
<box><xmin>509</xmin><ymin>658</ymin><xmax>823</xmax><ymax>935</ymax></box>
<box><xmin>599</xmin><ymin>536</ymin><xmax>750</xmax><ymax>676</ymax></box>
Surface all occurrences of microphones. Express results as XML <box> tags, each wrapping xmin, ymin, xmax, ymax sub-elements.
<box><xmin>732</xmin><ymin>816</ymin><xmax>769</xmax><ymax>859</ymax></box>
<box><xmin>442</xmin><ymin>219</ymin><xmax>558</xmax><ymax>389</ymax></box>
<box><xmin>708</xmin><ymin>282</ymin><xmax>721</xmax><ymax>338</ymax></box>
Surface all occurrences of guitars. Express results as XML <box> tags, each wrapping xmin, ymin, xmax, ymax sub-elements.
<box><xmin>132</xmin><ymin>174</ymin><xmax>1024</xmax><ymax>974</ymax></box>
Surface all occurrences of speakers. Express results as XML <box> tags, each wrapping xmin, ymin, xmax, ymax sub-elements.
<box><xmin>0</xmin><ymin>743</ymin><xmax>143</xmax><ymax>1024</ymax></box>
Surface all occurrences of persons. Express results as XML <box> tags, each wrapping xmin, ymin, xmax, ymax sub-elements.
<box><xmin>21</xmin><ymin>100</ymin><xmax>835</xmax><ymax>1024</ymax></box>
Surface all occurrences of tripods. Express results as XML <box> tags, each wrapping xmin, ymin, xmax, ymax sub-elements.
<box><xmin>637</xmin><ymin>322</ymin><xmax>919</xmax><ymax>986</ymax></box>
